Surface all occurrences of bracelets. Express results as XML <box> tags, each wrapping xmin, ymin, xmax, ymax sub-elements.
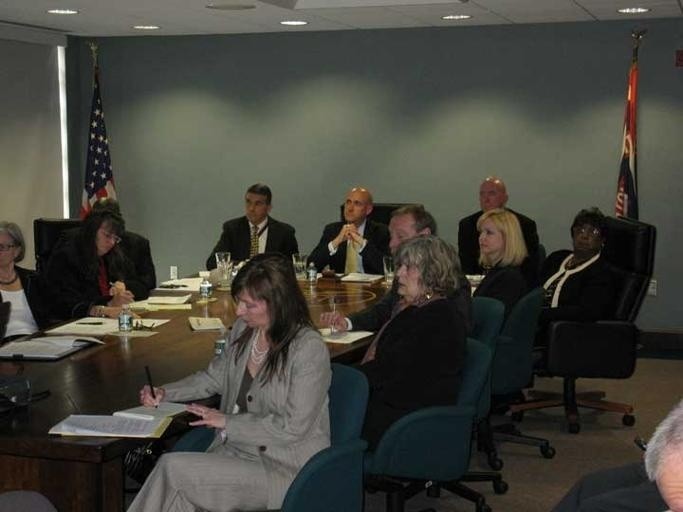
<box><xmin>93</xmin><ymin>303</ymin><xmax>106</xmax><ymax>317</ymax></box>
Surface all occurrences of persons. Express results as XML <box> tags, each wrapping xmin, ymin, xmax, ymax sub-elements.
<box><xmin>206</xmin><ymin>185</ymin><xmax>299</xmax><ymax>270</ymax></box>
<box><xmin>319</xmin><ymin>204</ymin><xmax>471</xmax><ymax>332</ymax></box>
<box><xmin>41</xmin><ymin>197</ymin><xmax>157</xmax><ymax>290</ymax></box>
<box><xmin>0</xmin><ymin>220</ymin><xmax>141</xmax><ymax>358</ymax></box>
<box><xmin>458</xmin><ymin>178</ymin><xmax>540</xmax><ymax>274</ymax></box>
<box><xmin>347</xmin><ymin>234</ymin><xmax>464</xmax><ymax>453</ymax></box>
<box><xmin>49</xmin><ymin>209</ymin><xmax>135</xmax><ymax>306</ymax></box>
<box><xmin>474</xmin><ymin>206</ymin><xmax>527</xmax><ymax>314</ymax></box>
<box><xmin>306</xmin><ymin>186</ymin><xmax>389</xmax><ymax>274</ymax></box>
<box><xmin>643</xmin><ymin>399</ymin><xmax>683</xmax><ymax>512</ymax></box>
<box><xmin>126</xmin><ymin>253</ymin><xmax>331</xmax><ymax>512</ymax></box>
<box><xmin>532</xmin><ymin>209</ymin><xmax>621</xmax><ymax>345</ymax></box>
<box><xmin>548</xmin><ymin>464</ymin><xmax>668</xmax><ymax>512</ymax></box>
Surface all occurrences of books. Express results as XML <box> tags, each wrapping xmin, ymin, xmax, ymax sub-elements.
<box><xmin>60</xmin><ymin>402</ymin><xmax>188</xmax><ymax>439</ymax></box>
<box><xmin>340</xmin><ymin>272</ymin><xmax>384</xmax><ymax>283</ymax></box>
<box><xmin>0</xmin><ymin>333</ymin><xmax>106</xmax><ymax>365</ymax></box>
<box><xmin>319</xmin><ymin>323</ymin><xmax>374</xmax><ymax>344</ymax></box>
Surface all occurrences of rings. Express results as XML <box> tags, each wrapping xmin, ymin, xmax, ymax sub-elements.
<box><xmin>201</xmin><ymin>408</ymin><xmax>205</xmax><ymax>413</ymax></box>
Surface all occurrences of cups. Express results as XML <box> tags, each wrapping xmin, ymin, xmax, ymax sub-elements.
<box><xmin>292</xmin><ymin>253</ymin><xmax>307</xmax><ymax>281</ymax></box>
<box><xmin>382</xmin><ymin>256</ymin><xmax>395</xmax><ymax>284</ymax></box>
<box><xmin>214</xmin><ymin>251</ymin><xmax>231</xmax><ymax>284</ymax></box>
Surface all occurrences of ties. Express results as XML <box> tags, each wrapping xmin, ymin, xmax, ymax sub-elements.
<box><xmin>250</xmin><ymin>228</ymin><xmax>259</xmax><ymax>257</ymax></box>
<box><xmin>345</xmin><ymin>240</ymin><xmax>356</xmax><ymax>274</ymax></box>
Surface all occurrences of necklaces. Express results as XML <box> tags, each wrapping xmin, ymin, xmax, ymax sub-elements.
<box><xmin>0</xmin><ymin>273</ymin><xmax>17</xmax><ymax>284</ymax></box>
<box><xmin>567</xmin><ymin>257</ymin><xmax>591</xmax><ymax>267</ymax></box>
<box><xmin>249</xmin><ymin>330</ymin><xmax>268</xmax><ymax>365</ymax></box>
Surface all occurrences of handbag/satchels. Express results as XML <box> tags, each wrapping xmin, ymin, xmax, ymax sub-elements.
<box><xmin>123</xmin><ymin>441</ymin><xmax>163</xmax><ymax>494</ymax></box>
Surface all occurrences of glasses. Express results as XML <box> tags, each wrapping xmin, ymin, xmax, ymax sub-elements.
<box><xmin>0</xmin><ymin>244</ymin><xmax>14</xmax><ymax>251</ymax></box>
<box><xmin>99</xmin><ymin>230</ymin><xmax>120</xmax><ymax>243</ymax></box>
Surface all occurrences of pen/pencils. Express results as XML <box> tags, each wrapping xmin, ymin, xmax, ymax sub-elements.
<box><xmin>145</xmin><ymin>366</ymin><xmax>157</xmax><ymax>408</ymax></box>
<box><xmin>76</xmin><ymin>322</ymin><xmax>102</xmax><ymax>325</ymax></box>
<box><xmin>109</xmin><ymin>282</ymin><xmax>135</xmax><ymax>304</ymax></box>
<box><xmin>331</xmin><ymin>304</ymin><xmax>336</xmax><ymax>335</ymax></box>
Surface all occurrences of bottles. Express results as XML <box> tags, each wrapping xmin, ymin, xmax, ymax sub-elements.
<box><xmin>200</xmin><ymin>277</ymin><xmax>212</xmax><ymax>297</ymax></box>
<box><xmin>307</xmin><ymin>261</ymin><xmax>318</xmax><ymax>282</ymax></box>
<box><xmin>118</xmin><ymin>304</ymin><xmax>133</xmax><ymax>332</ymax></box>
<box><xmin>214</xmin><ymin>328</ymin><xmax>226</xmax><ymax>355</ymax></box>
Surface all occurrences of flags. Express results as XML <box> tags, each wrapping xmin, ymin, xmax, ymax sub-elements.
<box><xmin>76</xmin><ymin>65</ymin><xmax>117</xmax><ymax>219</ymax></box>
<box><xmin>615</xmin><ymin>44</ymin><xmax>640</xmax><ymax>218</ymax></box>
